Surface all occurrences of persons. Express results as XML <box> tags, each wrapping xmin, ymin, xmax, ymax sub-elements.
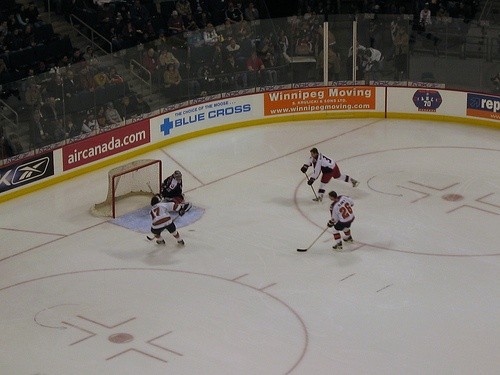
<box><xmin>301</xmin><ymin>148</ymin><xmax>358</xmax><ymax>202</ymax></box>
<box><xmin>325</xmin><ymin>191</ymin><xmax>355</xmax><ymax>249</ymax></box>
<box><xmin>160</xmin><ymin>170</ymin><xmax>190</xmax><ymax>216</ymax></box>
<box><xmin>150</xmin><ymin>197</ymin><xmax>187</xmax><ymax>246</ymax></box>
<box><xmin>1</xmin><ymin>0</ymin><xmax>500</xmax><ymax>166</ymax></box>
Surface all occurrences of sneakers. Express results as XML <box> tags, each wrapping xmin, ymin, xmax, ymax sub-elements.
<box><xmin>344</xmin><ymin>236</ymin><xmax>353</xmax><ymax>243</ymax></box>
<box><xmin>351</xmin><ymin>179</ymin><xmax>359</xmax><ymax>187</ymax></box>
<box><xmin>332</xmin><ymin>242</ymin><xmax>343</xmax><ymax>251</ymax></box>
<box><xmin>156</xmin><ymin>240</ymin><xmax>165</xmax><ymax>245</ymax></box>
<box><xmin>313</xmin><ymin>196</ymin><xmax>322</xmax><ymax>202</ymax></box>
<box><xmin>177</xmin><ymin>240</ymin><xmax>184</xmax><ymax>245</ymax></box>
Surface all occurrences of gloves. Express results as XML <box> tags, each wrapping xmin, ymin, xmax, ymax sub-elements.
<box><xmin>308</xmin><ymin>177</ymin><xmax>315</xmax><ymax>185</ymax></box>
<box><xmin>300</xmin><ymin>164</ymin><xmax>309</xmax><ymax>173</ymax></box>
<box><xmin>185</xmin><ymin>204</ymin><xmax>191</xmax><ymax>211</ymax></box>
<box><xmin>327</xmin><ymin>220</ymin><xmax>335</xmax><ymax>227</ymax></box>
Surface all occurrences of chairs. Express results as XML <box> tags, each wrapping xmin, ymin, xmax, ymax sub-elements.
<box><xmin>0</xmin><ymin>0</ymin><xmax>481</xmax><ymax>145</ymax></box>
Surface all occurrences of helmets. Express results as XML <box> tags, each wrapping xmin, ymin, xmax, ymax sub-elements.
<box><xmin>151</xmin><ymin>197</ymin><xmax>161</xmax><ymax>206</ymax></box>
<box><xmin>173</xmin><ymin>171</ymin><xmax>182</xmax><ymax>180</ymax></box>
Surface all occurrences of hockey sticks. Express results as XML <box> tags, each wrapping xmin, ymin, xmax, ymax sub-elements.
<box><xmin>146</xmin><ymin>214</ymin><xmax>180</xmax><ymax>241</ymax></box>
<box><xmin>296</xmin><ymin>226</ymin><xmax>329</xmax><ymax>253</ymax></box>
<box><xmin>146</xmin><ymin>182</ymin><xmax>155</xmax><ymax>196</ymax></box>
<box><xmin>304</xmin><ymin>172</ymin><xmax>319</xmax><ymax>201</ymax></box>
